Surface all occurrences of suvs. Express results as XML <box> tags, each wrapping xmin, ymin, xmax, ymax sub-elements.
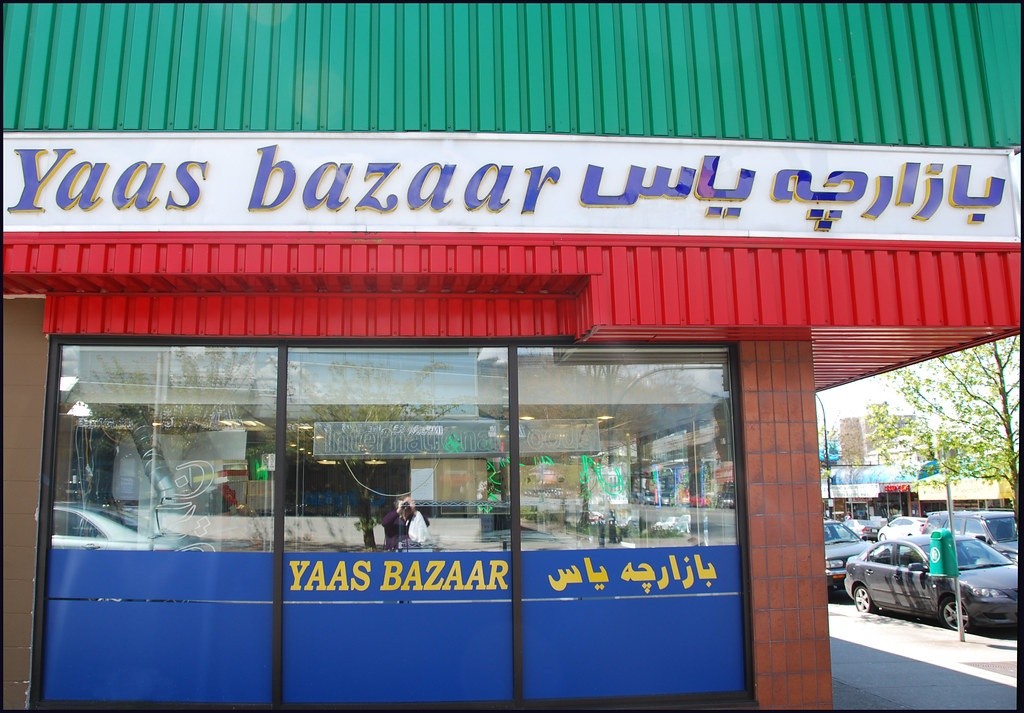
<box><xmin>920</xmin><ymin>510</ymin><xmax>1018</xmax><ymax>573</ymax></box>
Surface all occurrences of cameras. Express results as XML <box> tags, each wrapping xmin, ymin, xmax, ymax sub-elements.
<box><xmin>401</xmin><ymin>502</ymin><xmax>410</xmax><ymax>509</ymax></box>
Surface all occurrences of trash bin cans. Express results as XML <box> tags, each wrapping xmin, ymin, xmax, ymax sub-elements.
<box><xmin>928</xmin><ymin>528</ymin><xmax>959</xmax><ymax>578</ymax></box>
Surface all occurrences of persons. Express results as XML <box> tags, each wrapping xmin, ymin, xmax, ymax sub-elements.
<box><xmin>382</xmin><ymin>492</ymin><xmax>430</xmax><ymax>548</ymax></box>
<box><xmin>844</xmin><ymin>512</ymin><xmax>852</xmax><ymax>520</ymax></box>
<box><xmin>836</xmin><ymin>514</ymin><xmax>844</xmax><ymax>522</ymax></box>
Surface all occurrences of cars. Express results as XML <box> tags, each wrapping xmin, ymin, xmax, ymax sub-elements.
<box><xmin>566</xmin><ymin>512</ymin><xmax>604</xmax><ymax>533</ymax></box>
<box><xmin>876</xmin><ymin>516</ymin><xmax>928</xmax><ymax>544</ymax></box>
<box><xmin>823</xmin><ymin>516</ymin><xmax>875</xmax><ymax>598</ymax></box>
<box><xmin>521</xmin><ymin>486</ymin><xmax>569</xmax><ymax>499</ymax></box>
<box><xmin>835</xmin><ymin>519</ymin><xmax>881</xmax><ymax>543</ymax></box>
<box><xmin>46</xmin><ymin>500</ymin><xmax>219</xmax><ymax>551</ymax></box>
<box><xmin>842</xmin><ymin>534</ymin><xmax>1018</xmax><ymax>632</ymax></box>
<box><xmin>629</xmin><ymin>490</ymin><xmax>735</xmax><ymax>509</ymax></box>
